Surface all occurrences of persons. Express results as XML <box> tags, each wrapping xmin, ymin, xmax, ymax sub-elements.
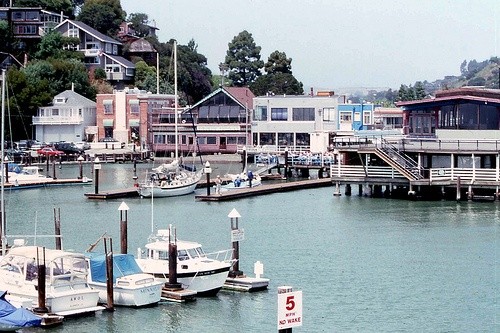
<box><xmin>215</xmin><ymin>175</ymin><xmax>222</xmax><ymax>194</ymax></box>
<box><xmin>248</xmin><ymin>172</ymin><xmax>253</xmax><ymax>187</ymax></box>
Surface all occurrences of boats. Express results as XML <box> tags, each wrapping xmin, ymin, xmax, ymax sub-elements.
<box><xmin>220</xmin><ymin>173</ymin><xmax>262</xmax><ymax>191</ymax></box>
<box><xmin>132</xmin><ymin>180</ymin><xmax>240</xmax><ymax>297</ymax></box>
<box><xmin>0</xmin><ymin>244</ymin><xmax>108</xmax><ymax>332</ymax></box>
<box><xmin>78</xmin><ymin>251</ymin><xmax>165</xmax><ymax>307</ymax></box>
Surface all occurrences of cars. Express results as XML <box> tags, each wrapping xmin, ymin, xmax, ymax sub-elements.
<box><xmin>0</xmin><ymin>139</ymin><xmax>91</xmax><ymax>163</ymax></box>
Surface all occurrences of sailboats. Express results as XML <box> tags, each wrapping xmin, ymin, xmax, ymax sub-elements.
<box><xmin>135</xmin><ymin>40</ymin><xmax>207</xmax><ymax>198</ymax></box>
